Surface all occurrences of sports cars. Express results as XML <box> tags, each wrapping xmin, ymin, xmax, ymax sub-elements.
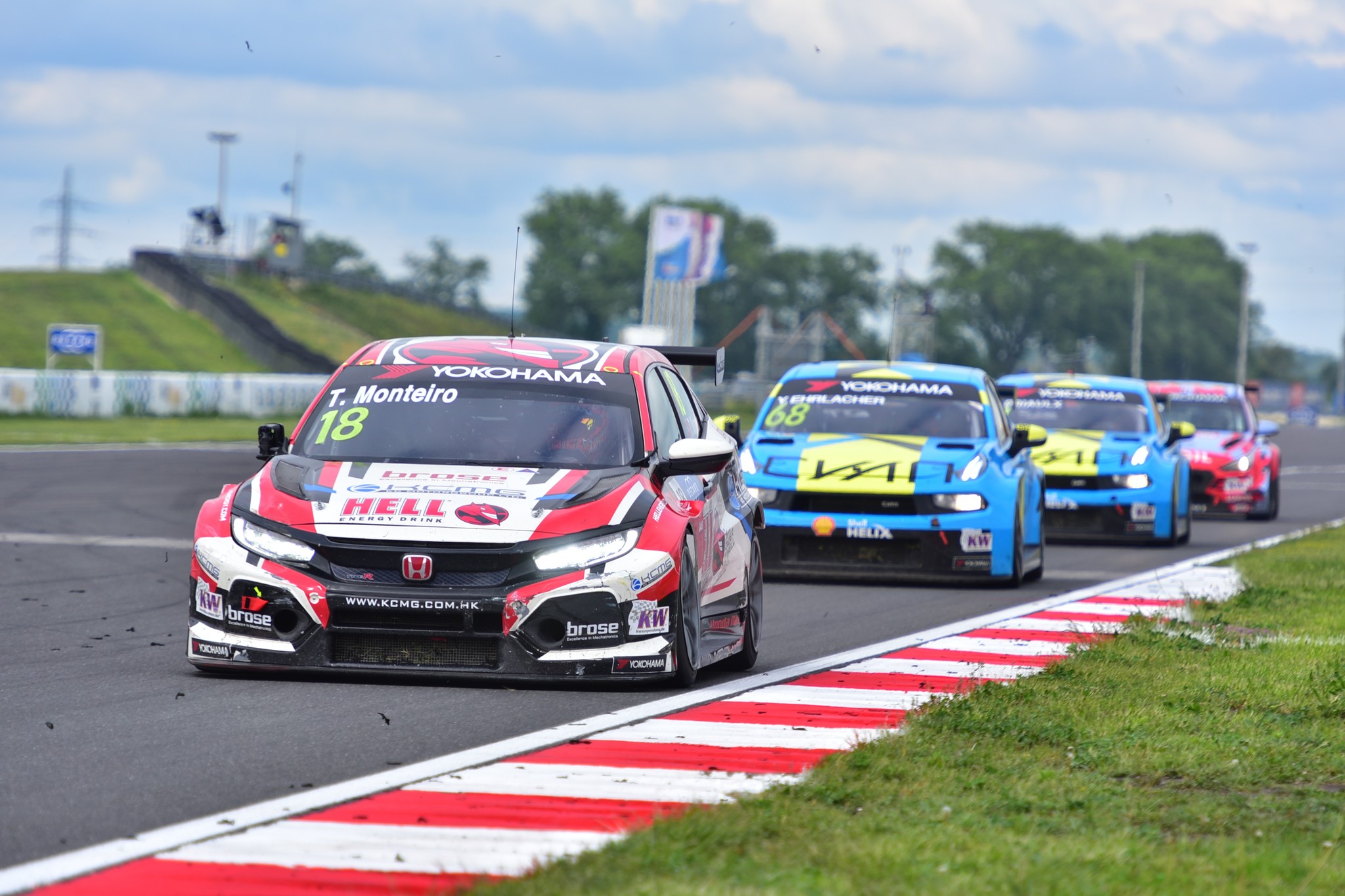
<box><xmin>714</xmin><ymin>359</ymin><xmax>1049</xmax><ymax>586</ymax></box>
<box><xmin>184</xmin><ymin>336</ymin><xmax>768</xmax><ymax>694</ymax></box>
<box><xmin>992</xmin><ymin>370</ymin><xmax>1198</xmax><ymax>542</ymax></box>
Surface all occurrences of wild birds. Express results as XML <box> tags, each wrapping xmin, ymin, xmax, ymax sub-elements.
<box><xmin>246</xmin><ymin>41</ymin><xmax>257</xmax><ymax>57</ymax></box>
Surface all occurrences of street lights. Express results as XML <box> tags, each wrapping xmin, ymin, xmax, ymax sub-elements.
<box><xmin>211</xmin><ymin>131</ymin><xmax>240</xmax><ymax>247</ymax></box>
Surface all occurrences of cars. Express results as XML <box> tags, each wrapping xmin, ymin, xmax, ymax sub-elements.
<box><xmin>1144</xmin><ymin>378</ymin><xmax>1284</xmax><ymax>521</ymax></box>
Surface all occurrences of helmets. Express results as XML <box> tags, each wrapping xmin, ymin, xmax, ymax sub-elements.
<box><xmin>544</xmin><ymin>391</ymin><xmax>608</xmax><ymax>456</ymax></box>
